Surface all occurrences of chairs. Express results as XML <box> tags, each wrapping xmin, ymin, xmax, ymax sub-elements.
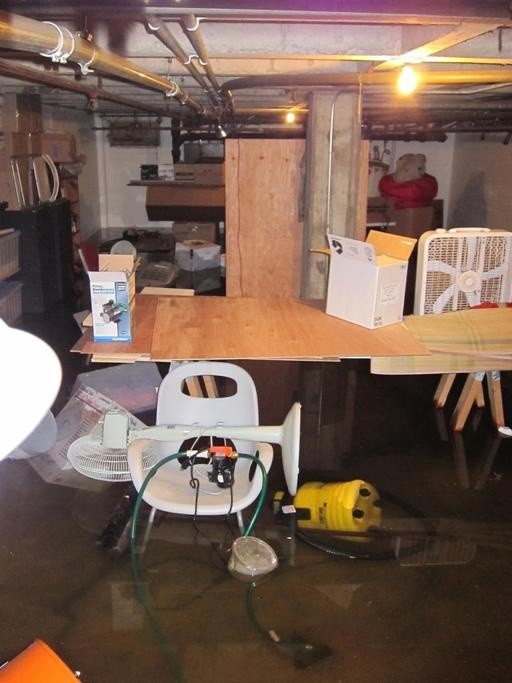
<box><xmin>125</xmin><ymin>362</ymin><xmax>274</xmax><ymax>542</ymax></box>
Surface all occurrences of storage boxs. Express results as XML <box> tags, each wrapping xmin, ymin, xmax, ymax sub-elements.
<box><xmin>172</xmin><ymin>238</ymin><xmax>221</xmax><ymax>271</ymax></box>
<box><xmin>139</xmin><ymin>155</ymin><xmax>226</xmax><ymax>184</ymax></box>
<box><xmin>308</xmin><ymin>227</ymin><xmax>419</xmax><ymax>329</ymax></box>
<box><xmin>87</xmin><ymin>252</ymin><xmax>137</xmax><ymax>343</ymax></box>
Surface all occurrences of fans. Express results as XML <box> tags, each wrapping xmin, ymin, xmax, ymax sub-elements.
<box><xmin>413</xmin><ymin>224</ymin><xmax>512</xmax><ymax>316</ymax></box>
<box><xmin>66</xmin><ymin>402</ymin><xmax>302</xmax><ymax>498</ymax></box>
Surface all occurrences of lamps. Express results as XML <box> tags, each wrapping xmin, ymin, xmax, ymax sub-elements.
<box><xmin>394</xmin><ymin>58</ymin><xmax>424</xmax><ymax>97</ymax></box>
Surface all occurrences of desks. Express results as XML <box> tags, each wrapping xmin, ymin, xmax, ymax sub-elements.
<box><xmin>89</xmin><ymin>287</ymin><xmax>511</xmax><ymax>433</ymax></box>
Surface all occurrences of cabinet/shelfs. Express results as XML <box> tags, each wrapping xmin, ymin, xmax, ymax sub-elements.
<box><xmin>218</xmin><ymin>138</ymin><xmax>308</xmax><ymax>297</ymax></box>
